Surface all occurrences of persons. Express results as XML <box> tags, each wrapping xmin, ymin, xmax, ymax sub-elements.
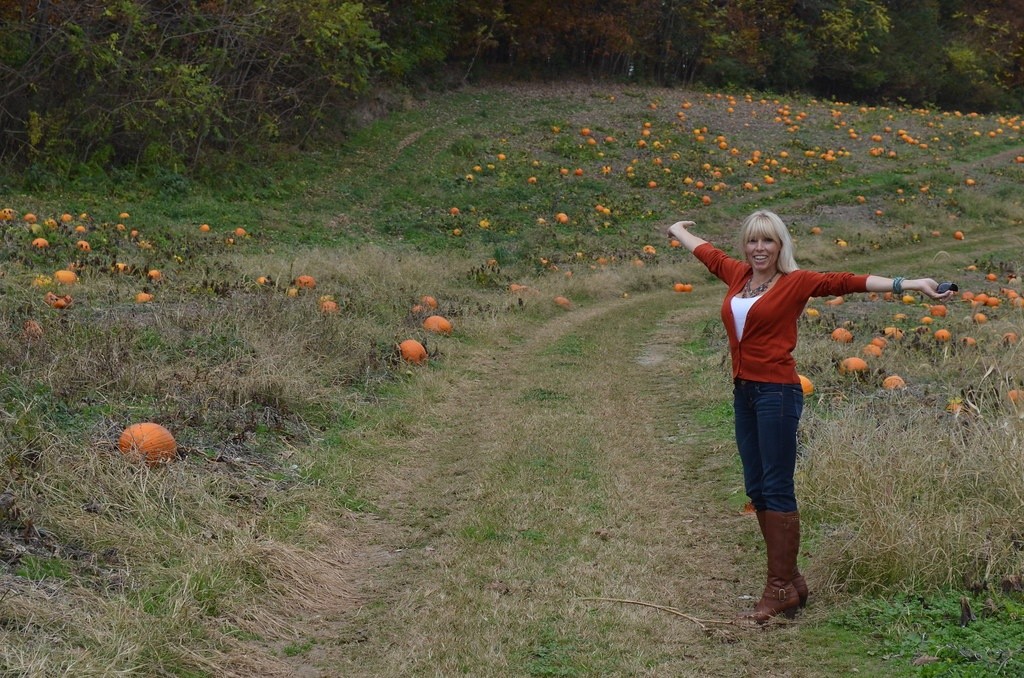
<box><xmin>667</xmin><ymin>211</ymin><xmax>956</xmax><ymax>622</ymax></box>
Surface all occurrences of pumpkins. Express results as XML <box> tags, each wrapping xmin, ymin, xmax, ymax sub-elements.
<box><xmin>119</xmin><ymin>422</ymin><xmax>177</xmax><ymax>469</ymax></box>
<box><xmin>398</xmin><ymin>93</ymin><xmax>1024</xmax><ymax>426</ymax></box>
<box><xmin>0</xmin><ymin>209</ymin><xmax>338</xmax><ymax>315</ymax></box>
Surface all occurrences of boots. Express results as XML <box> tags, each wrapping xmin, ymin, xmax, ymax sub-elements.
<box><xmin>755</xmin><ymin>507</ymin><xmax>809</xmax><ymax>608</ymax></box>
<box><xmin>740</xmin><ymin>509</ymin><xmax>801</xmax><ymax>629</ymax></box>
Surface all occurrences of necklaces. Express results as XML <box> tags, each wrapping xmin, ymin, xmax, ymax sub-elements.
<box><xmin>741</xmin><ymin>269</ymin><xmax>779</xmax><ymax>299</ymax></box>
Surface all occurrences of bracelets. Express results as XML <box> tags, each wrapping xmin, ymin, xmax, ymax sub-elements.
<box><xmin>893</xmin><ymin>277</ymin><xmax>906</xmax><ymax>295</ymax></box>
